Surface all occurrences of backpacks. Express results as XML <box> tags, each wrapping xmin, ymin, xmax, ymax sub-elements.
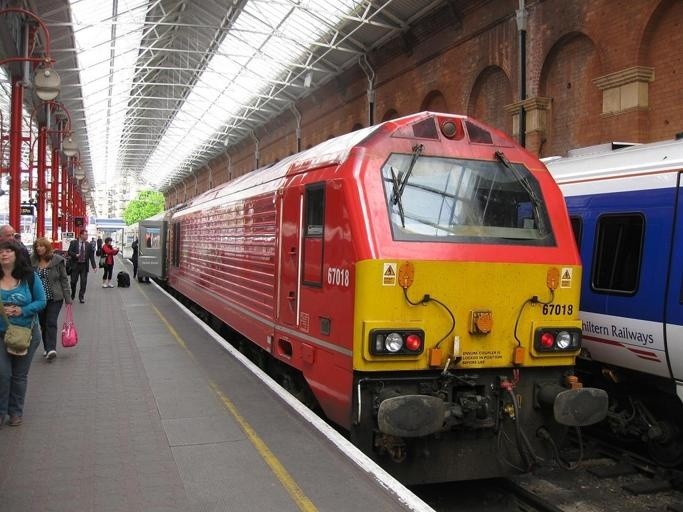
<box><xmin>117</xmin><ymin>272</ymin><xmax>130</xmax><ymax>287</ymax></box>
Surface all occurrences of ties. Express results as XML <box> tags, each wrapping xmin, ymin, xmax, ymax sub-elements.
<box><xmin>80</xmin><ymin>242</ymin><xmax>84</xmax><ymax>262</ymax></box>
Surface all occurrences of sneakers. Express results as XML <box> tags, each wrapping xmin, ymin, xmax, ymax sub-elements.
<box><xmin>102</xmin><ymin>284</ymin><xmax>113</xmax><ymax>288</ymax></box>
<box><xmin>43</xmin><ymin>350</ymin><xmax>56</xmax><ymax>361</ymax></box>
<box><xmin>79</xmin><ymin>293</ymin><xmax>85</xmax><ymax>303</ymax></box>
<box><xmin>71</xmin><ymin>294</ymin><xmax>75</xmax><ymax>299</ymax></box>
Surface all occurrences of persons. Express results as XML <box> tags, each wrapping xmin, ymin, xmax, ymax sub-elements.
<box><xmin>0</xmin><ymin>223</ymin><xmax>119</xmax><ymax>426</ymax></box>
<box><xmin>132</xmin><ymin>233</ymin><xmax>153</xmax><ymax>284</ymax></box>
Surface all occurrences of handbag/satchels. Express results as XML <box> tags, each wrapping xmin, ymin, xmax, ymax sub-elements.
<box><xmin>99</xmin><ymin>254</ymin><xmax>108</xmax><ymax>268</ymax></box>
<box><xmin>66</xmin><ymin>259</ymin><xmax>71</xmax><ymax>275</ymax></box>
<box><xmin>4</xmin><ymin>325</ymin><xmax>32</xmax><ymax>355</ymax></box>
<box><xmin>62</xmin><ymin>304</ymin><xmax>78</xmax><ymax>347</ymax></box>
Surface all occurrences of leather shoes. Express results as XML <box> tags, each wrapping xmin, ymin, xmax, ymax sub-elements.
<box><xmin>0</xmin><ymin>415</ymin><xmax>23</xmax><ymax>426</ymax></box>
<box><xmin>138</xmin><ymin>280</ymin><xmax>151</xmax><ymax>283</ymax></box>
